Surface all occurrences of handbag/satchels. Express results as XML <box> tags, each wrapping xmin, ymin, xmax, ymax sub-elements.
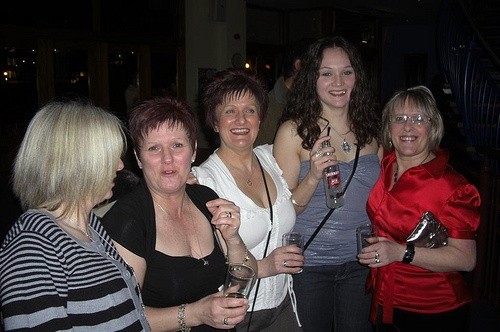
<box><xmin>407</xmin><ymin>212</ymin><xmax>449</xmax><ymax>250</ymax></box>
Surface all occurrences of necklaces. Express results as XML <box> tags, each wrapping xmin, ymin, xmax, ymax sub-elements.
<box><xmin>218</xmin><ymin>149</ymin><xmax>254</xmax><ymax>186</ymax></box>
<box><xmin>150</xmin><ymin>193</ymin><xmax>210</xmax><ymax>266</ymax></box>
<box><xmin>393</xmin><ymin>150</ymin><xmax>431</xmax><ymax>184</ymax></box>
<box><xmin>320</xmin><ymin>115</ymin><xmax>355</xmax><ymax>152</ymax></box>
<box><xmin>66</xmin><ymin>222</ymin><xmax>146</xmax><ymax>309</ymax></box>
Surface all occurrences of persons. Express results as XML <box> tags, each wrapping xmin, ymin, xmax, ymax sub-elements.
<box><xmin>365</xmin><ymin>82</ymin><xmax>482</xmax><ymax>332</ymax></box>
<box><xmin>0</xmin><ymin>99</ymin><xmax>153</xmax><ymax>332</ymax></box>
<box><xmin>270</xmin><ymin>34</ymin><xmax>387</xmax><ymax>332</ymax></box>
<box><xmin>183</xmin><ymin>69</ymin><xmax>305</xmax><ymax>332</ymax></box>
<box><xmin>251</xmin><ymin>39</ymin><xmax>311</xmax><ymax>150</ymax></box>
<box><xmin>99</xmin><ymin>97</ymin><xmax>259</xmax><ymax>332</ymax></box>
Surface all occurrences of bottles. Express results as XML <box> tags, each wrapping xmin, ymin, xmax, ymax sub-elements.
<box><xmin>323</xmin><ymin>140</ymin><xmax>344</xmax><ymax>209</ymax></box>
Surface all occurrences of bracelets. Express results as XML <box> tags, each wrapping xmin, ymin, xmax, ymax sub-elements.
<box><xmin>402</xmin><ymin>242</ymin><xmax>416</xmax><ymax>264</ymax></box>
<box><xmin>291</xmin><ymin>191</ymin><xmax>309</xmax><ymax>207</ymax></box>
<box><xmin>224</xmin><ymin>246</ymin><xmax>248</xmax><ymax>271</ymax></box>
<box><xmin>178</xmin><ymin>304</ymin><xmax>192</xmax><ymax>332</ymax></box>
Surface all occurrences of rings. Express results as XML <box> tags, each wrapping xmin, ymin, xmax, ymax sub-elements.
<box><xmin>376</xmin><ymin>259</ymin><xmax>380</xmax><ymax>264</ymax></box>
<box><xmin>223</xmin><ymin>318</ymin><xmax>228</xmax><ymax>325</ymax></box>
<box><xmin>227</xmin><ymin>211</ymin><xmax>232</xmax><ymax>218</ymax></box>
<box><xmin>284</xmin><ymin>260</ymin><xmax>287</xmax><ymax>267</ymax></box>
<box><xmin>315</xmin><ymin>151</ymin><xmax>322</xmax><ymax>158</ymax></box>
<box><xmin>374</xmin><ymin>251</ymin><xmax>379</xmax><ymax>259</ymax></box>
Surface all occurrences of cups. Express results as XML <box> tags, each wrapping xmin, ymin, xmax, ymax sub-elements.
<box><xmin>281</xmin><ymin>233</ymin><xmax>305</xmax><ymax>274</ymax></box>
<box><xmin>221</xmin><ymin>262</ymin><xmax>255</xmax><ymax>308</ymax></box>
<box><xmin>357</xmin><ymin>222</ymin><xmax>376</xmax><ymax>255</ymax></box>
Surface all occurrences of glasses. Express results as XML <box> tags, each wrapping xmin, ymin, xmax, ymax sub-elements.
<box><xmin>390</xmin><ymin>113</ymin><xmax>430</xmax><ymax>124</ymax></box>
